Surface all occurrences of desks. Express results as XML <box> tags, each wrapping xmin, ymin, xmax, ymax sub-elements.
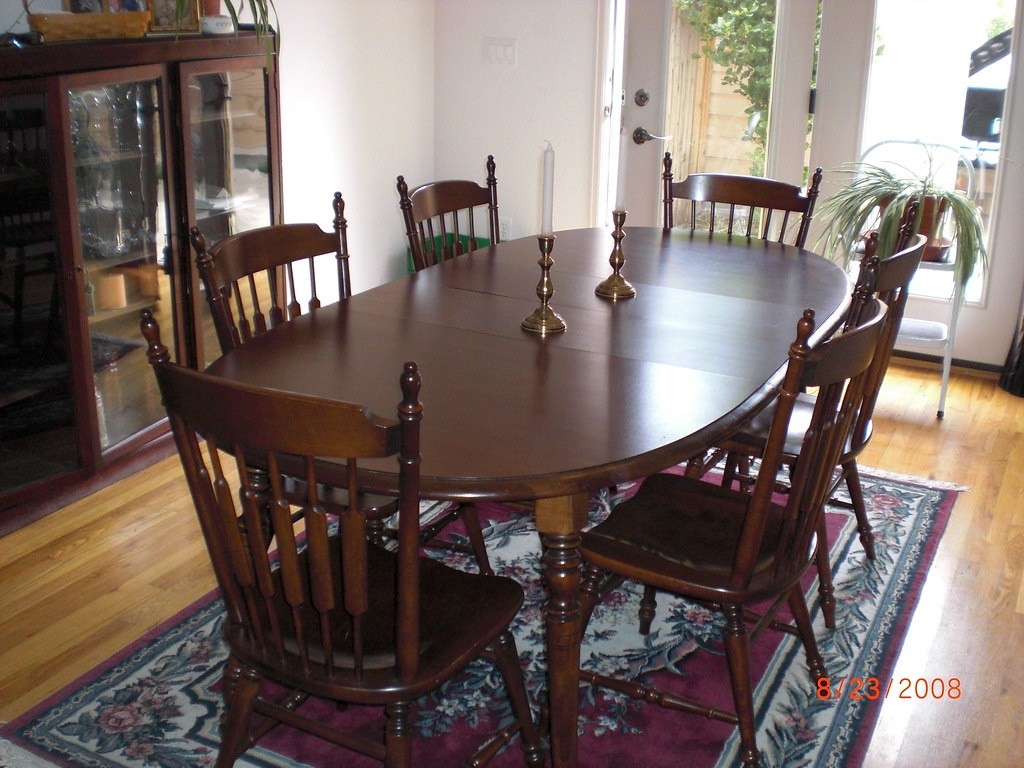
<box><xmin>178</xmin><ymin>227</ymin><xmax>850</xmax><ymax>767</ymax></box>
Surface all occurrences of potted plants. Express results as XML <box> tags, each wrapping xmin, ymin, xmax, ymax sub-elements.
<box><xmin>783</xmin><ymin>157</ymin><xmax>988</xmax><ymax>296</ymax></box>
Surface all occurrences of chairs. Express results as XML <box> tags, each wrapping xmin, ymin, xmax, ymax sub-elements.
<box><xmin>535</xmin><ymin>257</ymin><xmax>889</xmax><ymax>768</ymax></box>
<box><xmin>187</xmin><ymin>192</ymin><xmax>491</xmax><ymax>576</ymax></box>
<box><xmin>139</xmin><ymin>305</ymin><xmax>545</xmax><ymax>768</ymax></box>
<box><xmin>682</xmin><ymin>201</ymin><xmax>929</xmax><ymax>628</ymax></box>
<box><xmin>398</xmin><ymin>156</ymin><xmax>502</xmax><ymax>271</ymax></box>
<box><xmin>663</xmin><ymin>151</ymin><xmax>823</xmax><ymax>246</ymax></box>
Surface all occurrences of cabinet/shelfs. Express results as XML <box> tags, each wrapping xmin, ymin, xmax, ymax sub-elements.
<box><xmin>1</xmin><ymin>22</ymin><xmax>289</xmax><ymax>480</ymax></box>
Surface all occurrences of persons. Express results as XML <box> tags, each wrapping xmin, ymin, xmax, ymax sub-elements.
<box><xmin>156</xmin><ymin>0</ymin><xmax>180</xmax><ymax>26</ymax></box>
<box><xmin>109</xmin><ymin>0</ymin><xmax>128</xmax><ymax>14</ymax></box>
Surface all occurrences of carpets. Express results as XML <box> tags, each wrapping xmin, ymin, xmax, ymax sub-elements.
<box><xmin>0</xmin><ymin>446</ymin><xmax>960</xmax><ymax>767</ymax></box>
